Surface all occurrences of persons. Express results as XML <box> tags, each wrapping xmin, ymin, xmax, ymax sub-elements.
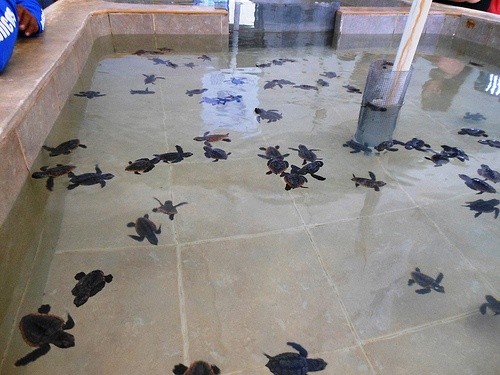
<box><xmin>0</xmin><ymin>0</ymin><xmax>45</xmax><ymax>74</ymax></box>
